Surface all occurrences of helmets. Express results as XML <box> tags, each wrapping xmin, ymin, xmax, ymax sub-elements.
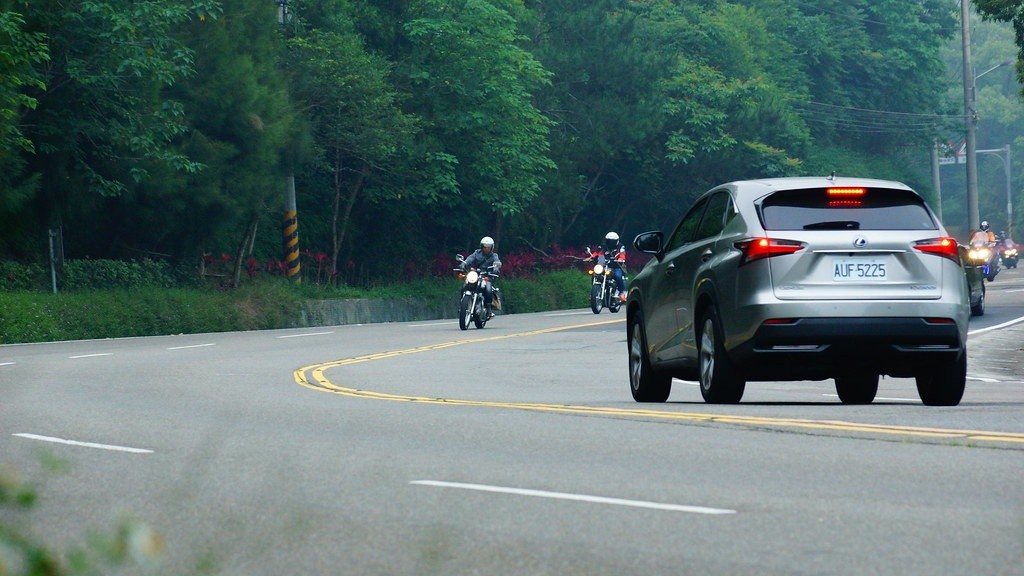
<box><xmin>605</xmin><ymin>231</ymin><xmax>620</xmax><ymax>251</ymax></box>
<box><xmin>979</xmin><ymin>220</ymin><xmax>990</xmax><ymax>233</ymax></box>
<box><xmin>479</xmin><ymin>236</ymin><xmax>494</xmax><ymax>255</ymax></box>
<box><xmin>999</xmin><ymin>231</ymin><xmax>1007</xmax><ymax>237</ymax></box>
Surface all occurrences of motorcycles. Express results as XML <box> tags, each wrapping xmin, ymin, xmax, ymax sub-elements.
<box><xmin>453</xmin><ymin>254</ymin><xmax>500</xmax><ymax>330</ymax></box>
<box><xmin>969</xmin><ymin>232</ymin><xmax>1001</xmax><ymax>281</ymax></box>
<box><xmin>583</xmin><ymin>247</ymin><xmax>628</xmax><ymax>314</ymax></box>
<box><xmin>999</xmin><ymin>239</ymin><xmax>1018</xmax><ymax>269</ymax></box>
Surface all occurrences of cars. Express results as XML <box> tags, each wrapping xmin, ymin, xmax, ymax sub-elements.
<box><xmin>956</xmin><ymin>241</ymin><xmax>985</xmax><ymax>316</ymax></box>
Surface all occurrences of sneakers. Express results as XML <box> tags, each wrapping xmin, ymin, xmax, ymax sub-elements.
<box><xmin>619</xmin><ymin>293</ymin><xmax>627</xmax><ymax>302</ymax></box>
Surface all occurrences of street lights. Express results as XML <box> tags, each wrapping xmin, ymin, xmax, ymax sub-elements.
<box><xmin>964</xmin><ymin>59</ymin><xmax>1013</xmax><ymax>240</ymax></box>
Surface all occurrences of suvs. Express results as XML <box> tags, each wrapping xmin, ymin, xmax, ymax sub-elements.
<box><xmin>625</xmin><ymin>171</ymin><xmax>971</xmax><ymax>406</ymax></box>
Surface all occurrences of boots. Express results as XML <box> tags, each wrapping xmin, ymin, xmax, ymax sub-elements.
<box><xmin>486</xmin><ymin>304</ymin><xmax>494</xmax><ymax>317</ymax></box>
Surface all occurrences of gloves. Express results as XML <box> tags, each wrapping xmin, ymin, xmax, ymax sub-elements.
<box><xmin>491</xmin><ymin>271</ymin><xmax>500</xmax><ymax>275</ymax></box>
<box><xmin>456</xmin><ymin>265</ymin><xmax>463</xmax><ymax>270</ymax></box>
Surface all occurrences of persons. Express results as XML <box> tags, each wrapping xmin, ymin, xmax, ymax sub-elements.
<box><xmin>456</xmin><ymin>236</ymin><xmax>500</xmax><ymax>316</ymax></box>
<box><xmin>585</xmin><ymin>231</ymin><xmax>627</xmax><ymax>302</ymax></box>
<box><xmin>969</xmin><ymin>221</ymin><xmax>996</xmax><ymax>273</ymax></box>
<box><xmin>997</xmin><ymin>231</ymin><xmax>1008</xmax><ymax>262</ymax></box>
